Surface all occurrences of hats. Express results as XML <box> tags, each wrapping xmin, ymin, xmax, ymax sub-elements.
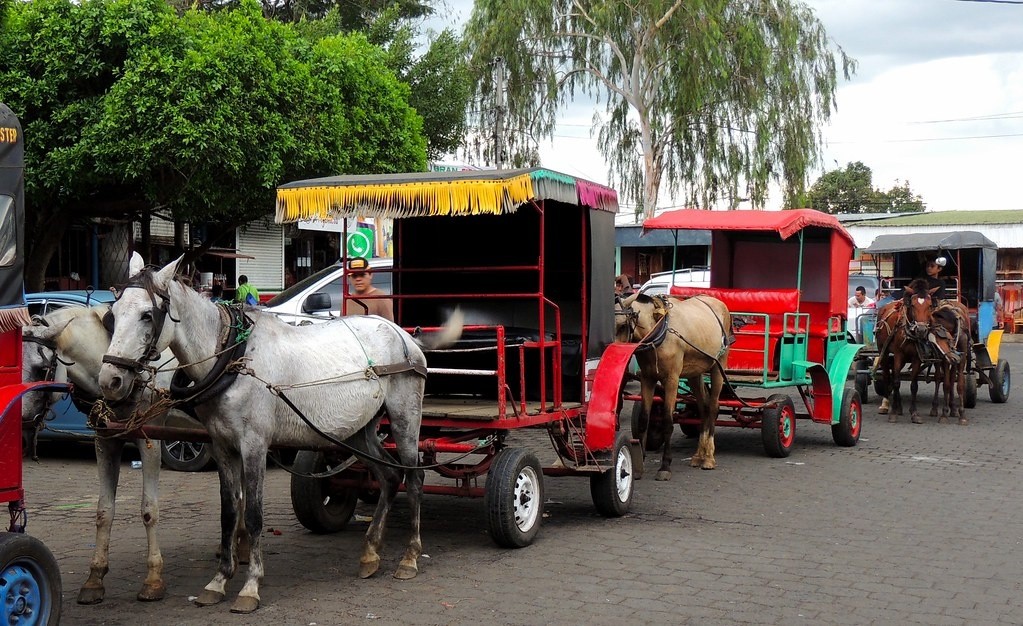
<box><xmin>347</xmin><ymin>257</ymin><xmax>370</xmax><ymax>273</ymax></box>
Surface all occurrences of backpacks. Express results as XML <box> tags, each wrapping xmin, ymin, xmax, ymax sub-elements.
<box><xmin>242</xmin><ymin>285</ymin><xmax>258</xmax><ymax>306</ymax></box>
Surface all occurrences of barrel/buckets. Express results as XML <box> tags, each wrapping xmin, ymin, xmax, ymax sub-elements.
<box><xmin>200</xmin><ymin>272</ymin><xmax>213</xmax><ymax>289</ymax></box>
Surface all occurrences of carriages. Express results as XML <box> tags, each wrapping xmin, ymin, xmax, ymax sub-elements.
<box><xmin>855</xmin><ymin>231</ymin><xmax>1011</xmax><ymax>425</ymax></box>
<box><xmin>23</xmin><ymin>167</ymin><xmax>648</xmax><ymax>615</ymax></box>
<box><xmin>615</xmin><ymin>208</ymin><xmax>867</xmax><ymax>480</ymax></box>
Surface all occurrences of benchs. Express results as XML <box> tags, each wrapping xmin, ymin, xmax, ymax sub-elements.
<box><xmin>793</xmin><ymin>300</ymin><xmax>830</xmax><ymax>361</ymax></box>
<box><xmin>962</xmin><ymin>288</ymin><xmax>977</xmax><ymax>332</ymax></box>
<box><xmin>436</xmin><ymin>299</ymin><xmax>585</xmax><ymax>400</ymax></box>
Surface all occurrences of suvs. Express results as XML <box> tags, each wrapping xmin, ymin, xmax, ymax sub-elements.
<box><xmin>848</xmin><ymin>274</ymin><xmax>891</xmax><ymax>299</ymax></box>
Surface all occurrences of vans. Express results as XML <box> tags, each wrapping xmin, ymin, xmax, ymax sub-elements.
<box><xmin>637</xmin><ymin>268</ymin><xmax>711</xmax><ymax>297</ymax></box>
<box><xmin>258</xmin><ymin>258</ymin><xmax>393</xmax><ymax>327</ymax></box>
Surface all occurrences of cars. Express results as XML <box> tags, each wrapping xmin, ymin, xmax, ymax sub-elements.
<box><xmin>24</xmin><ymin>290</ymin><xmax>213</xmax><ymax>473</ymax></box>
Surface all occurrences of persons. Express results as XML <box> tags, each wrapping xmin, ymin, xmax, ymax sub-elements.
<box><xmin>993</xmin><ymin>292</ymin><xmax>1004</xmax><ymax>330</ymax></box>
<box><xmin>235</xmin><ymin>275</ymin><xmax>260</xmax><ymax>306</ymax></box>
<box><xmin>340</xmin><ymin>257</ymin><xmax>395</xmax><ymax>324</ymax></box>
<box><xmin>891</xmin><ymin>254</ymin><xmax>948</xmax><ymax>304</ymax></box>
<box><xmin>210</xmin><ymin>285</ymin><xmax>224</xmax><ymax>304</ymax></box>
<box><xmin>848</xmin><ymin>284</ymin><xmax>891</xmax><ymax>317</ymax></box>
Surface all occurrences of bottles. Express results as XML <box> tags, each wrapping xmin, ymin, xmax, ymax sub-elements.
<box><xmin>214</xmin><ymin>274</ymin><xmax>227</xmax><ymax>288</ymax></box>
<box><xmin>131</xmin><ymin>461</ymin><xmax>142</xmax><ymax>469</ymax></box>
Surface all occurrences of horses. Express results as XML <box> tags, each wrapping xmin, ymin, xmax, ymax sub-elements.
<box><xmin>875</xmin><ymin>279</ymin><xmax>943</xmax><ymax>423</ymax></box>
<box><xmin>99</xmin><ymin>249</ymin><xmax>462</xmax><ymax>615</ymax></box>
<box><xmin>929</xmin><ymin>300</ymin><xmax>973</xmax><ymax>424</ymax></box>
<box><xmin>613</xmin><ymin>291</ymin><xmax>731</xmax><ymax>480</ymax></box>
<box><xmin>19</xmin><ymin>305</ymin><xmax>249</xmax><ymax>608</ymax></box>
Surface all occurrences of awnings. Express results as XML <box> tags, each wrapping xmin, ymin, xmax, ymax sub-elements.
<box><xmin>142</xmin><ymin>237</ymin><xmax>256</xmax><ymax>260</ymax></box>
